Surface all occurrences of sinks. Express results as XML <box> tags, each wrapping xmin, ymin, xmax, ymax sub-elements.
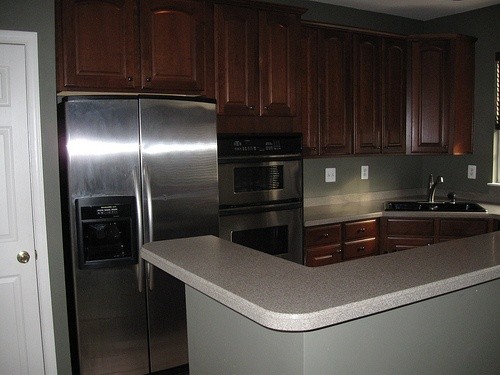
<box><xmin>428</xmin><ymin>201</ymin><xmax>487</xmax><ymax>212</ymax></box>
<box><xmin>383</xmin><ymin>201</ymin><xmax>437</xmax><ymax>213</ymax></box>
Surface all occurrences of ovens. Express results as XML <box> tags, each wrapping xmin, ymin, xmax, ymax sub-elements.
<box><xmin>216</xmin><ymin>132</ymin><xmax>304</xmax><ymax>266</ymax></box>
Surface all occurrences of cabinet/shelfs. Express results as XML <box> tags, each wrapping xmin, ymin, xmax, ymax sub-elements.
<box><xmin>303</xmin><ymin>217</ymin><xmax>384</xmax><ymax>267</ymax></box>
<box><xmin>301</xmin><ymin>18</ymin><xmax>355</xmax><ymax>158</ymax></box>
<box><xmin>407</xmin><ymin>32</ymin><xmax>479</xmax><ymax>157</ymax></box>
<box><xmin>384</xmin><ymin>217</ymin><xmax>490</xmax><ymax>254</ymax></box>
<box><xmin>217</xmin><ymin>0</ymin><xmax>309</xmax><ymax>116</ymax></box>
<box><xmin>54</xmin><ymin>0</ymin><xmax>215</xmax><ymax>99</ymax></box>
<box><xmin>355</xmin><ymin>27</ymin><xmax>406</xmax><ymax>156</ymax></box>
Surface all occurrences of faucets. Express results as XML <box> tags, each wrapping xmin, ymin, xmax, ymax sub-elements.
<box><xmin>427</xmin><ymin>173</ymin><xmax>444</xmax><ymax>203</ymax></box>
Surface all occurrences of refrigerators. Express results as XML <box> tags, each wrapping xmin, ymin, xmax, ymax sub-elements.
<box><xmin>57</xmin><ymin>93</ymin><xmax>221</xmax><ymax>375</ymax></box>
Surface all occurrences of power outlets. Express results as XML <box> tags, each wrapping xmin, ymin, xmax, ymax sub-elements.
<box><xmin>467</xmin><ymin>164</ymin><xmax>477</xmax><ymax>180</ymax></box>
<box><xmin>324</xmin><ymin>167</ymin><xmax>337</xmax><ymax>183</ymax></box>
<box><xmin>360</xmin><ymin>165</ymin><xmax>369</xmax><ymax>180</ymax></box>
